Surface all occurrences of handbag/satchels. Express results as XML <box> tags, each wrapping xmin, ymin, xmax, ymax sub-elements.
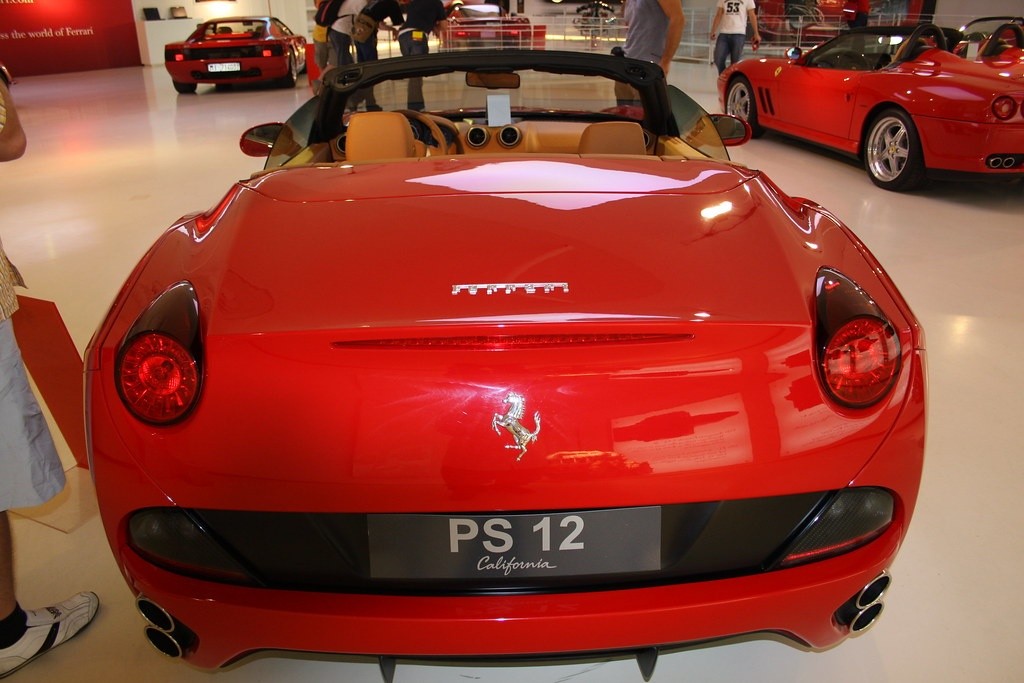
<box><xmin>5</xmin><ymin>296</ymin><xmax>102</xmax><ymax>535</ymax></box>
<box><xmin>350</xmin><ymin>13</ymin><xmax>378</xmax><ymax>43</ymax></box>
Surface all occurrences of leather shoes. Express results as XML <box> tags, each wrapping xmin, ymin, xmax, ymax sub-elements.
<box><xmin>0</xmin><ymin>591</ymin><xmax>99</xmax><ymax>678</ymax></box>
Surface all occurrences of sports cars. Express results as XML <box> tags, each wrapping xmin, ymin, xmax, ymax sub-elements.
<box><xmin>748</xmin><ymin>0</ymin><xmax>870</xmax><ymax>49</ymax></box>
<box><xmin>439</xmin><ymin>0</ymin><xmax>547</xmax><ymax>50</ymax></box>
<box><xmin>79</xmin><ymin>47</ymin><xmax>930</xmax><ymax>683</ymax></box>
<box><xmin>164</xmin><ymin>15</ymin><xmax>307</xmax><ymax>93</ymax></box>
<box><xmin>716</xmin><ymin>16</ymin><xmax>1024</xmax><ymax>190</ymax></box>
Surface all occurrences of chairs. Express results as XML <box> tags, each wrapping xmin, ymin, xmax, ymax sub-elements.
<box><xmin>215</xmin><ymin>27</ymin><xmax>233</xmax><ymax>33</ymax></box>
<box><xmin>578</xmin><ymin>122</ymin><xmax>647</xmax><ymax>154</ymax></box>
<box><xmin>890</xmin><ymin>23</ymin><xmax>1024</xmax><ymax>63</ymax></box>
<box><xmin>345</xmin><ymin>112</ymin><xmax>414</xmax><ymax>164</ymax></box>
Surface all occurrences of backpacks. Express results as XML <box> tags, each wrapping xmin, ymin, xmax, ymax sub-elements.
<box><xmin>314</xmin><ymin>1</ymin><xmax>344</xmax><ymax>27</ymax></box>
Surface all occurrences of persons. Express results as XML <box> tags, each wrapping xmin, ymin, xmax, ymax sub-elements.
<box><xmin>313</xmin><ymin>0</ymin><xmax>344</xmax><ymax>73</ymax></box>
<box><xmin>710</xmin><ymin>0</ymin><xmax>761</xmax><ymax>76</ymax></box>
<box><xmin>346</xmin><ymin>0</ymin><xmax>404</xmax><ymax>110</ymax></box>
<box><xmin>615</xmin><ymin>0</ymin><xmax>685</xmax><ymax>108</ymax></box>
<box><xmin>0</xmin><ymin>64</ymin><xmax>98</xmax><ymax>677</ymax></box>
<box><xmin>330</xmin><ymin>0</ymin><xmax>368</xmax><ymax>67</ymax></box>
<box><xmin>399</xmin><ymin>0</ymin><xmax>463</xmax><ymax>112</ymax></box>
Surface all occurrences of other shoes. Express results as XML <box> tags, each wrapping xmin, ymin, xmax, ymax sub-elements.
<box><xmin>365</xmin><ymin>104</ymin><xmax>382</xmax><ymax>112</ymax></box>
